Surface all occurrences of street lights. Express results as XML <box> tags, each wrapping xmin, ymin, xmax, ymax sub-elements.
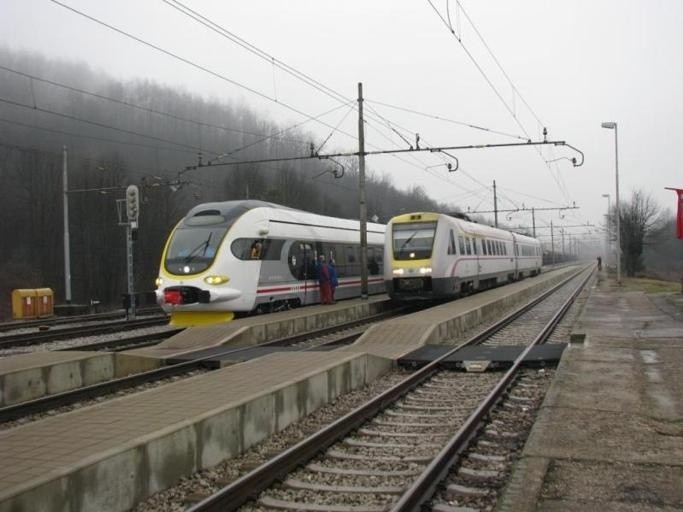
<box><xmin>601</xmin><ymin>121</ymin><xmax>621</xmax><ymax>281</ymax></box>
<box><xmin>602</xmin><ymin>194</ymin><xmax>612</xmax><ymax>243</ymax></box>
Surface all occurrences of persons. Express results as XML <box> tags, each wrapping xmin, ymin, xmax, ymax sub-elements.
<box><xmin>597</xmin><ymin>255</ymin><xmax>601</xmax><ymax>271</ymax></box>
<box><xmin>251</xmin><ymin>242</ymin><xmax>261</xmax><ymax>259</ymax></box>
<box><xmin>319</xmin><ymin>255</ymin><xmax>339</xmax><ymax>305</ymax></box>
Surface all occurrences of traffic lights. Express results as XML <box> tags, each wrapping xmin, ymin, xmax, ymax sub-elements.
<box><xmin>126</xmin><ymin>185</ymin><xmax>140</xmax><ymax>221</ymax></box>
